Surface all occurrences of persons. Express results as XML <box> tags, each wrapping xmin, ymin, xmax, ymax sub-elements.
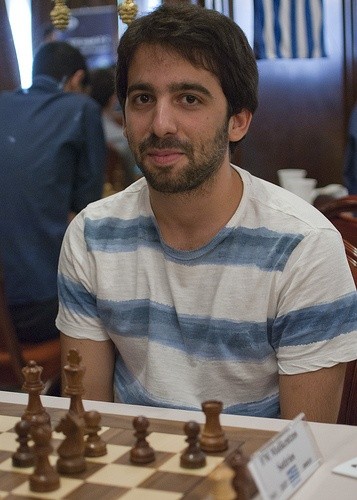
<box><xmin>0</xmin><ymin>39</ymin><xmax>109</xmax><ymax>351</ymax></box>
<box><xmin>54</xmin><ymin>2</ymin><xmax>357</xmax><ymax>426</ymax></box>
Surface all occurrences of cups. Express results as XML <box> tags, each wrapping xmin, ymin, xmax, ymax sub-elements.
<box><xmin>277</xmin><ymin>168</ymin><xmax>316</xmax><ymax>205</ymax></box>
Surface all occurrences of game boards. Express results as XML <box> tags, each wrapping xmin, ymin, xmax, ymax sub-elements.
<box><xmin>0</xmin><ymin>402</ymin><xmax>282</xmax><ymax>500</ymax></box>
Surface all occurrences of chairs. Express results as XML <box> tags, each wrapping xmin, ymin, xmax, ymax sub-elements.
<box><xmin>0</xmin><ymin>274</ymin><xmax>63</xmax><ymax>397</ymax></box>
<box><xmin>314</xmin><ymin>195</ymin><xmax>357</xmax><ymax>427</ymax></box>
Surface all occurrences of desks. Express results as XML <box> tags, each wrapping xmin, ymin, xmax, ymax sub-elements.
<box><xmin>2</xmin><ymin>391</ymin><xmax>357</xmax><ymax>500</ymax></box>
